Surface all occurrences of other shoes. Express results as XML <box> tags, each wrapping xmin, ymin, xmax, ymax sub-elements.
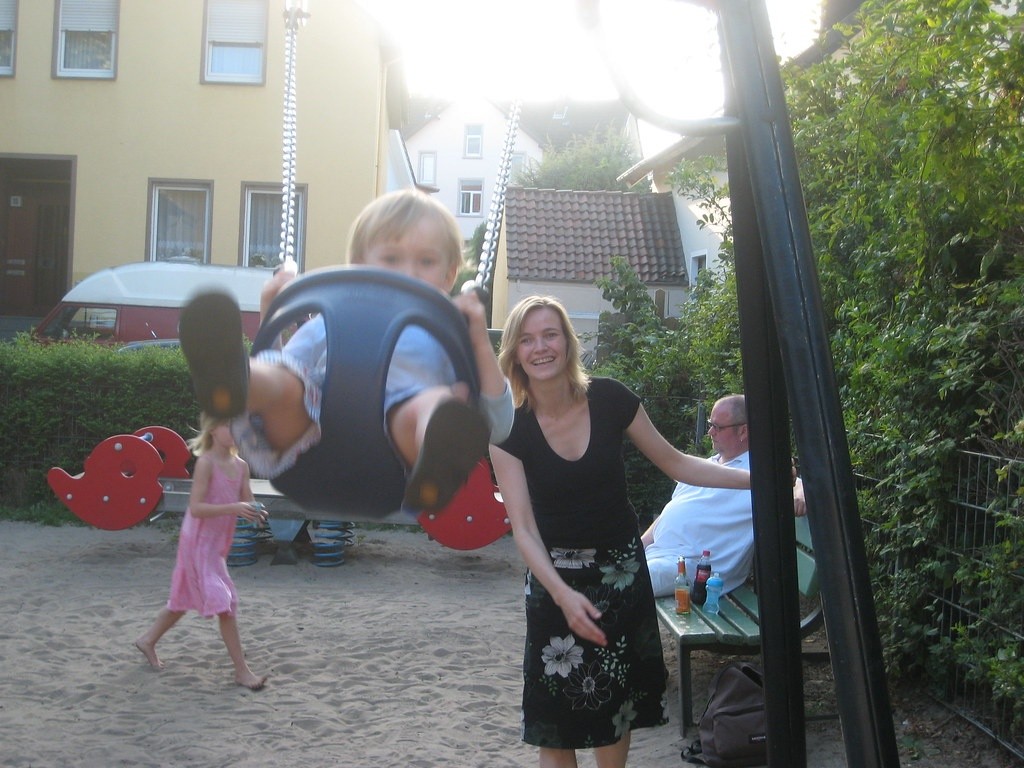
<box><xmin>178</xmin><ymin>293</ymin><xmax>251</xmax><ymax>420</ymax></box>
<box><xmin>407</xmin><ymin>394</ymin><xmax>491</xmax><ymax>514</ymax></box>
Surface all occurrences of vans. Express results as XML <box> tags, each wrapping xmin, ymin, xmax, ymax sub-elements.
<box><xmin>32</xmin><ymin>261</ymin><xmax>277</xmax><ymax>353</ymax></box>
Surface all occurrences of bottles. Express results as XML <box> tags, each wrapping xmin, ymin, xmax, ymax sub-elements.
<box><xmin>691</xmin><ymin>550</ymin><xmax>711</xmax><ymax>605</ymax></box>
<box><xmin>702</xmin><ymin>573</ymin><xmax>723</xmax><ymax>614</ymax></box>
<box><xmin>675</xmin><ymin>555</ymin><xmax>690</xmax><ymax>616</ymax></box>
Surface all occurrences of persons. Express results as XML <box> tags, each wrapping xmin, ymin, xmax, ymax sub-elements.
<box><xmin>133</xmin><ymin>409</ymin><xmax>272</xmax><ymax>691</ymax></box>
<box><xmin>175</xmin><ymin>185</ymin><xmax>517</xmax><ymax>516</ymax></box>
<box><xmin>630</xmin><ymin>394</ymin><xmax>808</xmax><ymax>730</ymax></box>
<box><xmin>488</xmin><ymin>292</ymin><xmax>798</xmax><ymax>768</ymax></box>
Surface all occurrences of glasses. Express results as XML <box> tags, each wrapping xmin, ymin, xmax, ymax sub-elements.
<box><xmin>707</xmin><ymin>420</ymin><xmax>746</xmax><ymax>433</ymax></box>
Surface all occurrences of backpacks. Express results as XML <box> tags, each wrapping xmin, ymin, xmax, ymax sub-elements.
<box><xmin>679</xmin><ymin>660</ymin><xmax>767</xmax><ymax>768</ymax></box>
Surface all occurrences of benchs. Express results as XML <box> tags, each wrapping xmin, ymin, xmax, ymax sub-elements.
<box><xmin>654</xmin><ymin>513</ymin><xmax>826</xmax><ymax>736</ymax></box>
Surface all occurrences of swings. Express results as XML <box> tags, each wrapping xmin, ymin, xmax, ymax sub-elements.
<box><xmin>245</xmin><ymin>0</ymin><xmax>544</xmax><ymax>522</ymax></box>
